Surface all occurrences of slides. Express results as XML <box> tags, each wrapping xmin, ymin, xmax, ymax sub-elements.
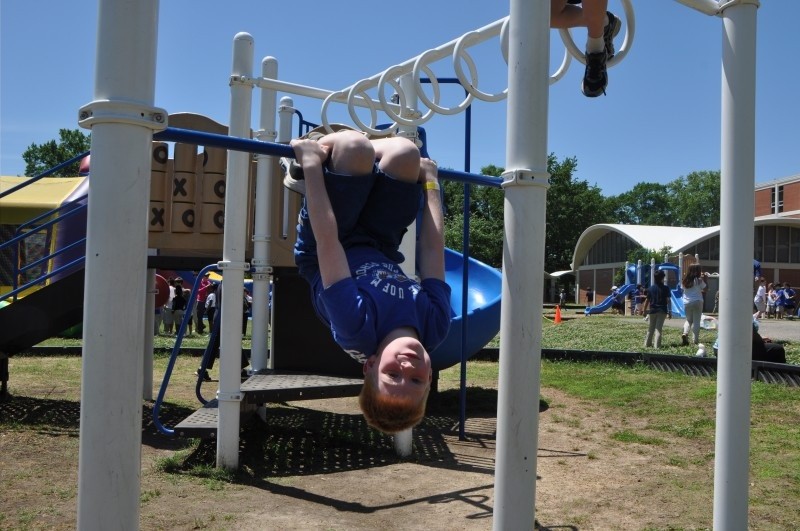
<box><xmin>584</xmin><ymin>283</ymin><xmax>636</xmax><ymax>316</ymax></box>
<box><xmin>433</xmin><ymin>245</ymin><xmax>501</xmax><ymax>376</ymax></box>
<box><xmin>666</xmin><ymin>286</ymin><xmax>688</xmax><ymax>318</ymax></box>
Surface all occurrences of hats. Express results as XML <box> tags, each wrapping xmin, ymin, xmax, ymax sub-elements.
<box><xmin>205</xmin><ymin>273</ymin><xmax>210</xmax><ymax>277</ymax></box>
<box><xmin>610</xmin><ymin>286</ymin><xmax>617</xmax><ymax>290</ymax></box>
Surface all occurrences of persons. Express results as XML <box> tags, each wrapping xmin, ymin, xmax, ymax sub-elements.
<box><xmin>610</xmin><ymin>283</ymin><xmax>624</xmax><ymax>317</ymax></box>
<box><xmin>160</xmin><ymin>271</ymin><xmax>272</xmax><ymax>339</ymax></box>
<box><xmin>634</xmin><ymin>283</ymin><xmax>650</xmax><ymax>317</ymax></box>
<box><xmin>681</xmin><ymin>263</ymin><xmax>708</xmax><ymax>345</ymax></box>
<box><xmin>754</xmin><ymin>260</ymin><xmax>761</xmax><ymax>282</ymax></box>
<box><xmin>712</xmin><ymin>289</ymin><xmax>719</xmax><ymax>314</ymax></box>
<box><xmin>752</xmin><ymin>279</ymin><xmax>795</xmax><ymax>321</ymax></box>
<box><xmin>558</xmin><ymin>289</ymin><xmax>567</xmax><ymax>312</ymax></box>
<box><xmin>279</xmin><ymin>128</ymin><xmax>458</xmax><ymax>431</ymax></box>
<box><xmin>585</xmin><ymin>286</ymin><xmax>593</xmax><ymax>307</ymax></box>
<box><xmin>642</xmin><ymin>270</ymin><xmax>672</xmax><ymax>350</ymax></box>
<box><xmin>712</xmin><ymin>308</ymin><xmax>787</xmax><ymax>363</ymax></box>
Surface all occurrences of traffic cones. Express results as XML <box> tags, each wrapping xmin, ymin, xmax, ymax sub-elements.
<box><xmin>554</xmin><ymin>305</ymin><xmax>562</xmax><ymax>323</ymax></box>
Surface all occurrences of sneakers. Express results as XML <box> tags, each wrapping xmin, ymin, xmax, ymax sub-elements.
<box><xmin>581</xmin><ymin>47</ymin><xmax>609</xmax><ymax>98</ymax></box>
<box><xmin>279</xmin><ymin>156</ymin><xmax>307</xmax><ymax>195</ymax></box>
<box><xmin>605</xmin><ymin>12</ymin><xmax>621</xmax><ymax>61</ymax></box>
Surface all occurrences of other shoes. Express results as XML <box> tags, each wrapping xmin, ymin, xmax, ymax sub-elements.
<box><xmin>680</xmin><ymin>334</ymin><xmax>689</xmax><ymax>345</ymax></box>
